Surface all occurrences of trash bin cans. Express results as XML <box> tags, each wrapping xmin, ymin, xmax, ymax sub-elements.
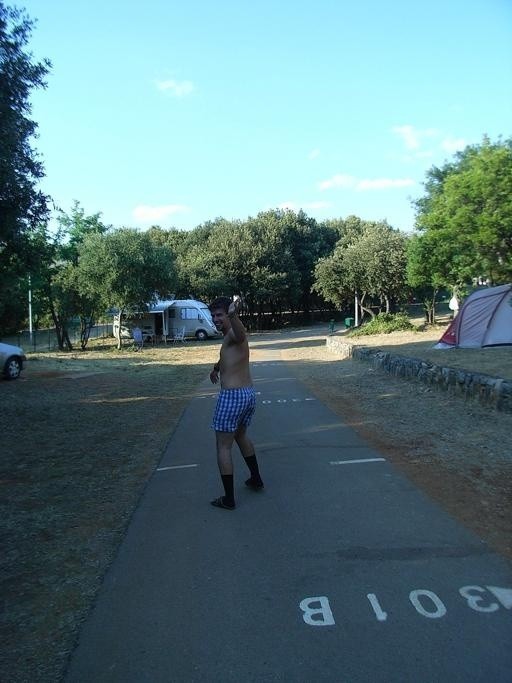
<box><xmin>345</xmin><ymin>318</ymin><xmax>354</xmax><ymax>329</ymax></box>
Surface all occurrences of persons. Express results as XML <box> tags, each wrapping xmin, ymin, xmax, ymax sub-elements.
<box><xmin>207</xmin><ymin>296</ymin><xmax>265</xmax><ymax>511</ymax></box>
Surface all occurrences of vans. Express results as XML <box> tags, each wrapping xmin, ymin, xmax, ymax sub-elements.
<box><xmin>113</xmin><ymin>299</ymin><xmax>226</xmax><ymax>341</ymax></box>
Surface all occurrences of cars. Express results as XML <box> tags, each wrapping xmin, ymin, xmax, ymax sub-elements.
<box><xmin>0</xmin><ymin>342</ymin><xmax>27</xmax><ymax>380</ymax></box>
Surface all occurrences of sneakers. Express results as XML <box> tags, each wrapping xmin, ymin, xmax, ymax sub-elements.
<box><xmin>245</xmin><ymin>477</ymin><xmax>264</xmax><ymax>488</ymax></box>
<box><xmin>209</xmin><ymin>496</ymin><xmax>236</xmax><ymax>510</ymax></box>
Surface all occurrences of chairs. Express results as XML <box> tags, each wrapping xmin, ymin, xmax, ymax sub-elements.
<box><xmin>121</xmin><ymin>326</ymin><xmax>187</xmax><ymax>353</ymax></box>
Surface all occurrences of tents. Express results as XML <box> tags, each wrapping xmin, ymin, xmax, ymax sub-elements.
<box><xmin>433</xmin><ymin>280</ymin><xmax>512</xmax><ymax>351</ymax></box>
